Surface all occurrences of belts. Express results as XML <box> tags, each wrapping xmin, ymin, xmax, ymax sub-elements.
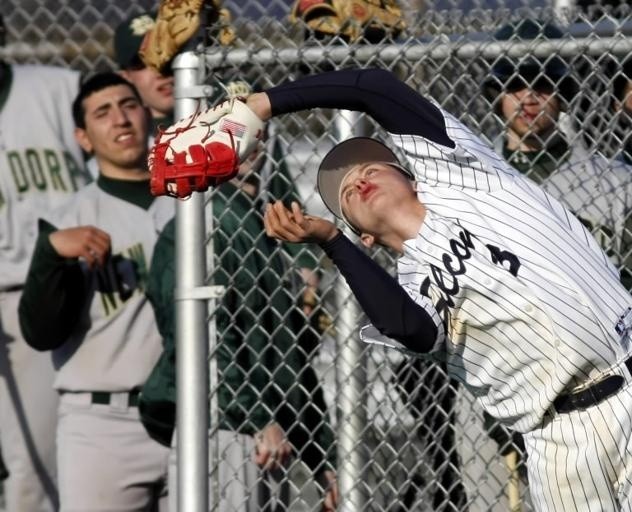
<box><xmin>90</xmin><ymin>390</ymin><xmax>143</xmax><ymax>407</ymax></box>
<box><xmin>552</xmin><ymin>354</ymin><xmax>631</xmax><ymax>414</ymax></box>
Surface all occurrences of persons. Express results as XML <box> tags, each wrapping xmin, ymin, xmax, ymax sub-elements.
<box><xmin>19</xmin><ymin>67</ymin><xmax>174</xmax><ymax>510</ymax></box>
<box><xmin>448</xmin><ymin>22</ymin><xmax>631</xmax><ymax>512</ymax></box>
<box><xmin>0</xmin><ymin>18</ymin><xmax>94</xmax><ymax>511</ymax></box>
<box><xmin>113</xmin><ymin>12</ymin><xmax>303</xmax><ymax>216</ymax></box>
<box><xmin>139</xmin><ymin>79</ymin><xmax>345</xmax><ymax>512</ymax></box>
<box><xmin>145</xmin><ymin>57</ymin><xmax>630</xmax><ymax>512</ymax></box>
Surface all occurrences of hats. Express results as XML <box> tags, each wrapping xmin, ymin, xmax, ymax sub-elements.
<box><xmin>482</xmin><ymin>17</ymin><xmax>584</xmax><ymax>116</ymax></box>
<box><xmin>315</xmin><ymin>137</ymin><xmax>416</xmax><ymax>237</ymax></box>
<box><xmin>113</xmin><ymin>11</ymin><xmax>158</xmax><ymax>70</ymax></box>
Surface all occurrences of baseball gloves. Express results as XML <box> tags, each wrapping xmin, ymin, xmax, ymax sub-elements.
<box><xmin>147</xmin><ymin>96</ymin><xmax>269</xmax><ymax>202</ymax></box>
<box><xmin>289</xmin><ymin>1</ymin><xmax>404</xmax><ymax>42</ymax></box>
<box><xmin>138</xmin><ymin>1</ymin><xmax>237</xmax><ymax>76</ymax></box>
